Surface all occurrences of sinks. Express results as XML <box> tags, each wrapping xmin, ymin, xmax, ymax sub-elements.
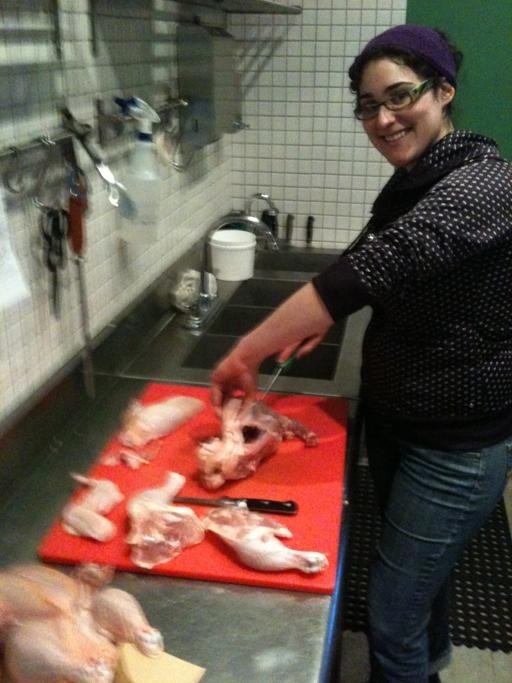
<box><xmin>259</xmin><ymin>247</ymin><xmax>347</xmax><ymax>274</ymax></box>
<box><xmin>207</xmin><ymin>304</ymin><xmax>348</xmax><ymax>346</ymax></box>
<box><xmin>180</xmin><ymin>330</ymin><xmax>345</xmax><ymax>379</ymax></box>
<box><xmin>230</xmin><ymin>277</ymin><xmax>308</xmax><ymax>307</ymax></box>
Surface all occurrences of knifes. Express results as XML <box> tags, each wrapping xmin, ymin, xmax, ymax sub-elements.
<box><xmin>259</xmin><ymin>349</ymin><xmax>301</xmax><ymax>401</ymax></box>
<box><xmin>167</xmin><ymin>490</ymin><xmax>299</xmax><ymax>516</ymax></box>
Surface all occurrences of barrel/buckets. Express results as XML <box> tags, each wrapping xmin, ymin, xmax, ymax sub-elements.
<box><xmin>206</xmin><ymin>229</ymin><xmax>259</xmax><ymax>284</ymax></box>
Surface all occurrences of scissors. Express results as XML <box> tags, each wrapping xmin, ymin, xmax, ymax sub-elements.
<box><xmin>38</xmin><ymin>205</ymin><xmax>72</xmax><ymax>322</ymax></box>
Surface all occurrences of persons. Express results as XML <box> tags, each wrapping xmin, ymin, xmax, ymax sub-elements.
<box><xmin>209</xmin><ymin>25</ymin><xmax>511</xmax><ymax>683</ymax></box>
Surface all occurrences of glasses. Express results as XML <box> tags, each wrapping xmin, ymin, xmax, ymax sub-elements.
<box><xmin>354</xmin><ymin>77</ymin><xmax>447</xmax><ymax>120</ymax></box>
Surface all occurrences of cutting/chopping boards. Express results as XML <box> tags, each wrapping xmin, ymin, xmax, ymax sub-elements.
<box><xmin>35</xmin><ymin>382</ymin><xmax>347</xmax><ymax>595</ymax></box>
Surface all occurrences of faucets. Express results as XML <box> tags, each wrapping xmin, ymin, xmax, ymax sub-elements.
<box><xmin>243</xmin><ymin>192</ymin><xmax>282</xmax><ymax>221</ymax></box>
<box><xmin>203</xmin><ymin>212</ymin><xmax>282</xmax><ymax>270</ymax></box>
